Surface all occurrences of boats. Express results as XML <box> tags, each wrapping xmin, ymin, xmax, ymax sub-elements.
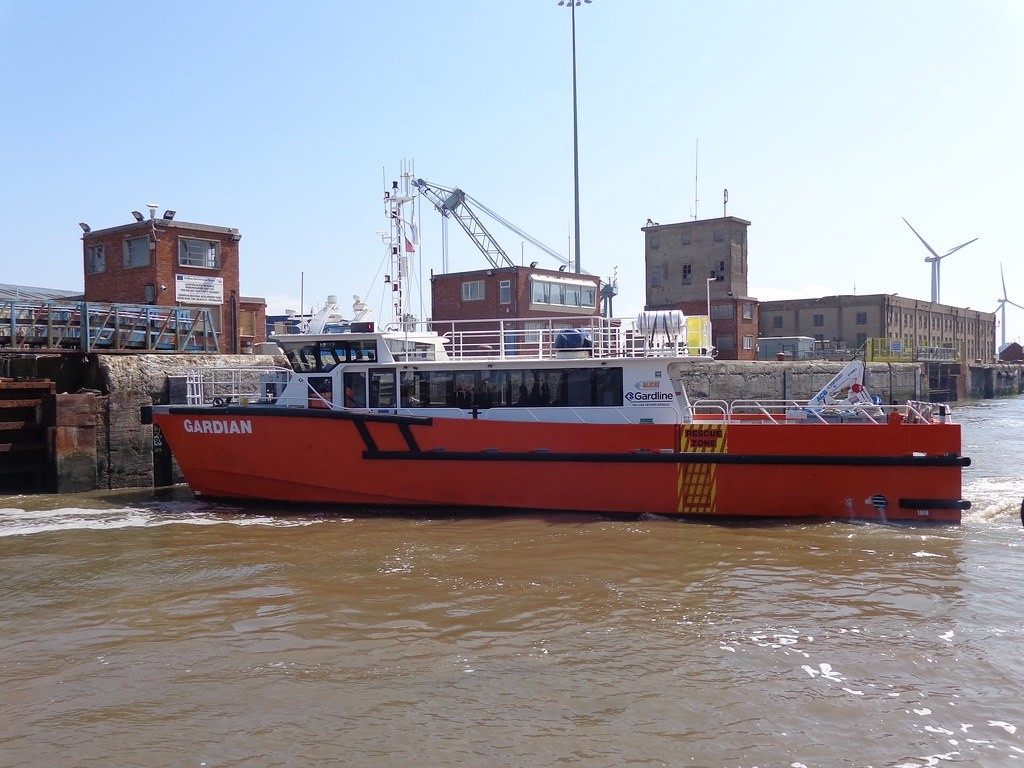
<box><xmin>139</xmin><ymin>294</ymin><xmax>974</xmax><ymax>523</ymax></box>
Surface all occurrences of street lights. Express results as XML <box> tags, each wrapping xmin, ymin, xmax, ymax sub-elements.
<box><xmin>707</xmin><ymin>277</ymin><xmax>718</xmax><ymax>316</ymax></box>
<box><xmin>556</xmin><ymin>0</ymin><xmax>595</xmax><ymax>274</ymax></box>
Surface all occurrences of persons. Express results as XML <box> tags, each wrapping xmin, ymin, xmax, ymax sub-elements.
<box><xmin>512</xmin><ymin>383</ymin><xmax>550</xmax><ymax>407</ymax></box>
<box><xmin>352</xmin><ymin>351</ymin><xmax>375</xmax><ymax>361</ymax></box>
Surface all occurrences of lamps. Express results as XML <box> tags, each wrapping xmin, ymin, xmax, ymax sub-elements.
<box><xmin>163</xmin><ymin>210</ymin><xmax>176</xmax><ymax>220</ymax></box>
<box><xmin>131</xmin><ymin>211</ymin><xmax>145</xmax><ymax>222</ymax></box>
<box><xmin>78</xmin><ymin>221</ymin><xmax>91</xmax><ymax>232</ymax></box>
<box><xmin>486</xmin><ymin>270</ymin><xmax>493</xmax><ymax>276</ymax></box>
<box><xmin>231</xmin><ymin>234</ymin><xmax>242</xmax><ymax>243</ymax></box>
<box><xmin>530</xmin><ymin>261</ymin><xmax>538</xmax><ymax>267</ymax></box>
<box><xmin>559</xmin><ymin>264</ymin><xmax>566</xmax><ymax>272</ymax></box>
<box><xmin>728</xmin><ymin>291</ymin><xmax>733</xmax><ymax>296</ymax></box>
